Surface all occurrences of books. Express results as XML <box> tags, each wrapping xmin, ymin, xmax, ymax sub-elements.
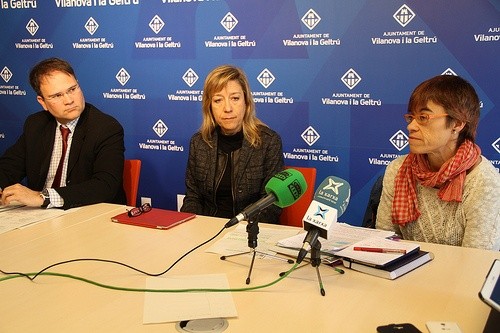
<box><xmin>340</xmin><ymin>238</ymin><xmax>434</xmax><ymax>279</ymax></box>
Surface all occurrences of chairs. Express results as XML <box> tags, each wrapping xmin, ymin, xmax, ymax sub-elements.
<box><xmin>280</xmin><ymin>167</ymin><xmax>316</xmax><ymax>228</ymax></box>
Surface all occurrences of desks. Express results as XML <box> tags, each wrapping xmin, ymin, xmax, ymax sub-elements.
<box><xmin>0</xmin><ymin>202</ymin><xmax>500</xmax><ymax>333</ymax></box>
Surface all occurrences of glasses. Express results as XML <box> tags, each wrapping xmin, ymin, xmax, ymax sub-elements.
<box><xmin>41</xmin><ymin>81</ymin><xmax>80</xmax><ymax>102</ymax></box>
<box><xmin>126</xmin><ymin>203</ymin><xmax>152</xmax><ymax>217</ymax></box>
<box><xmin>404</xmin><ymin>114</ymin><xmax>449</xmax><ymax>125</ymax></box>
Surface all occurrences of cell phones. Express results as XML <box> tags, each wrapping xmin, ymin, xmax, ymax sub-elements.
<box><xmin>479</xmin><ymin>258</ymin><xmax>500</xmax><ymax>312</ymax></box>
<box><xmin>376</xmin><ymin>323</ymin><xmax>422</xmax><ymax>333</ymax></box>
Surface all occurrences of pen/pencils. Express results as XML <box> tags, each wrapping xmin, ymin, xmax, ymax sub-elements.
<box><xmin>353</xmin><ymin>246</ymin><xmax>407</xmax><ymax>255</ymax></box>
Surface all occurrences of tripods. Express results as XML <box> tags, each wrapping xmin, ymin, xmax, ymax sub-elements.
<box><xmin>279</xmin><ymin>239</ymin><xmax>345</xmax><ymax>296</ymax></box>
<box><xmin>220</xmin><ymin>211</ymin><xmax>294</xmax><ymax>285</ymax></box>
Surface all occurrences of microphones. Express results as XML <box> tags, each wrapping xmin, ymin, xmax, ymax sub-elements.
<box><xmin>224</xmin><ymin>169</ymin><xmax>307</xmax><ymax>228</ymax></box>
<box><xmin>296</xmin><ymin>176</ymin><xmax>351</xmax><ymax>265</ymax></box>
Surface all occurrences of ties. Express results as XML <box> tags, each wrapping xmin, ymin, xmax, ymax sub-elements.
<box><xmin>51</xmin><ymin>125</ymin><xmax>71</xmax><ymax>188</ymax></box>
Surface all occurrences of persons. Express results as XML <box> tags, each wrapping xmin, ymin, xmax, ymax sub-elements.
<box><xmin>181</xmin><ymin>67</ymin><xmax>284</xmax><ymax>225</ymax></box>
<box><xmin>375</xmin><ymin>76</ymin><xmax>500</xmax><ymax>250</ymax></box>
<box><xmin>0</xmin><ymin>58</ymin><xmax>127</xmax><ymax>209</ymax></box>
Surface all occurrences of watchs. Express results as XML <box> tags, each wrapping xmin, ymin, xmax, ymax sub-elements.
<box><xmin>40</xmin><ymin>188</ymin><xmax>50</xmax><ymax>207</ymax></box>
